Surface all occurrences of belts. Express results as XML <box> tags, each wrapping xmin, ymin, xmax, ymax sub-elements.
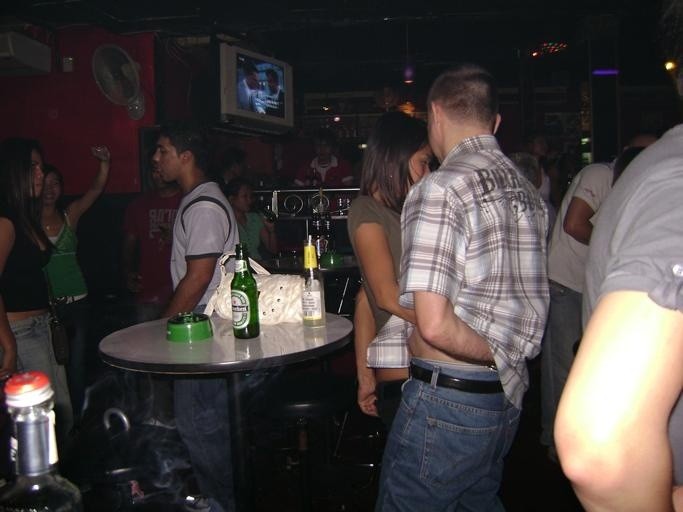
<box><xmin>410</xmin><ymin>360</ymin><xmax>505</xmax><ymax>393</ymax></box>
<box><xmin>54</xmin><ymin>293</ymin><xmax>87</xmax><ymax>305</ymax></box>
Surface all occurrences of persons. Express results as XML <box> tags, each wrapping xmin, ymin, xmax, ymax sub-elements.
<box><xmin>0</xmin><ymin>118</ymin><xmax>278</xmax><ymax>512</ymax></box>
<box><xmin>347</xmin><ymin>68</ymin><xmax>682</xmax><ymax>510</ymax></box>
<box><xmin>237</xmin><ymin>66</ymin><xmax>284</xmax><ymax>118</ymax></box>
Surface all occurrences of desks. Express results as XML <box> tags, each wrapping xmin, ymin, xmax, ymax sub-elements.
<box><xmin>98</xmin><ymin>310</ymin><xmax>353</xmax><ymax>511</ymax></box>
<box><xmin>263</xmin><ymin>252</ymin><xmax>360</xmax><ymax>316</ymax></box>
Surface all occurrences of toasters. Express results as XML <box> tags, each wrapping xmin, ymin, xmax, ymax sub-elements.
<box><xmin>1</xmin><ymin>369</ymin><xmax>84</xmax><ymax>512</ymax></box>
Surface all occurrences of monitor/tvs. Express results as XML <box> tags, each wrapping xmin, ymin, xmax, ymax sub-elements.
<box><xmin>220</xmin><ymin>44</ymin><xmax>295</xmax><ymax>129</ymax></box>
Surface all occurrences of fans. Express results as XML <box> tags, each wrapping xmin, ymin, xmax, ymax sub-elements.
<box><xmin>91</xmin><ymin>44</ymin><xmax>145</xmax><ymax>119</ymax></box>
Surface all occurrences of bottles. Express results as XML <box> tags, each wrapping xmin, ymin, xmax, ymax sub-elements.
<box><xmin>311</xmin><ymin>211</ymin><xmax>335</xmax><ymax>262</ymax></box>
<box><xmin>302</xmin><ymin>326</ymin><xmax>328</xmax><ymax>349</ymax></box>
<box><xmin>233</xmin><ymin>335</ymin><xmax>262</xmax><ymax>364</ymax></box>
<box><xmin>228</xmin><ymin>241</ymin><xmax>261</xmax><ymax>341</ymax></box>
<box><xmin>300</xmin><ymin>233</ymin><xmax>329</xmax><ymax>328</ymax></box>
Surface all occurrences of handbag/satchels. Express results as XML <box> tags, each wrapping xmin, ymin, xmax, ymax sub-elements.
<box><xmin>51</xmin><ymin>319</ymin><xmax>69</xmax><ymax>365</ymax></box>
<box><xmin>214</xmin><ymin>273</ymin><xmax>305</xmax><ymax>325</ymax></box>
